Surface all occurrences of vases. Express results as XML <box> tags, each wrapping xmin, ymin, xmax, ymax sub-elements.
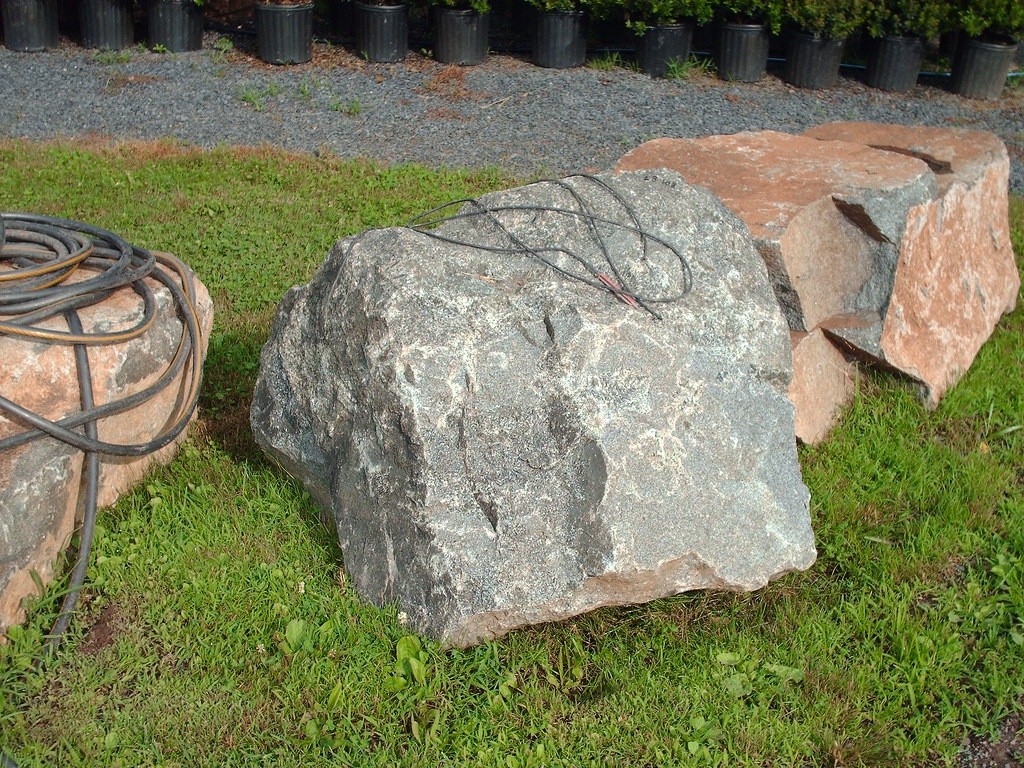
<box><xmin>0</xmin><ymin>0</ymin><xmax>59</xmax><ymax>53</ymax></box>
<box><xmin>147</xmin><ymin>0</ymin><xmax>203</xmax><ymax>52</ymax></box>
<box><xmin>79</xmin><ymin>0</ymin><xmax>134</xmax><ymax>50</ymax></box>
<box><xmin>353</xmin><ymin>1</ymin><xmax>407</xmax><ymax>63</ymax></box>
<box><xmin>253</xmin><ymin>2</ymin><xmax>312</xmax><ymax>65</ymax></box>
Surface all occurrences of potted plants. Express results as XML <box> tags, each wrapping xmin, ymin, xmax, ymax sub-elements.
<box><xmin>431</xmin><ymin>0</ymin><xmax>1024</xmax><ymax>102</ymax></box>
